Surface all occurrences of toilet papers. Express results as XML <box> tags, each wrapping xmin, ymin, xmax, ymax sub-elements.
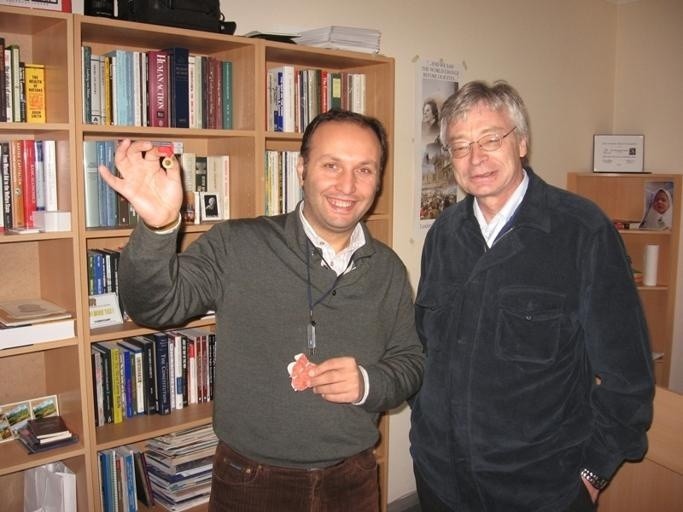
<box><xmin>642</xmin><ymin>245</ymin><xmax>660</xmax><ymax>286</ymax></box>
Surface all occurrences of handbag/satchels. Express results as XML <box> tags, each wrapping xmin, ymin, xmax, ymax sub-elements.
<box><xmin>86</xmin><ymin>1</ymin><xmax>236</xmax><ymax>34</ymax></box>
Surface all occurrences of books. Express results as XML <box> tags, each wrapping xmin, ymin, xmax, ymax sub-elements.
<box><xmin>2</xmin><ymin>38</ymin><xmax>58</xmax><ymax>230</ymax></box>
<box><xmin>87</xmin><ymin>245</ymin><xmax>216</xmax><ymax>427</ymax></box>
<box><xmin>265</xmin><ymin>66</ymin><xmax>366</xmax><ymax>216</ymax></box>
<box><xmin>98</xmin><ymin>424</ymin><xmax>219</xmax><ymax>512</ymax></box>
<box><xmin>82</xmin><ymin>46</ymin><xmax>231</xmax><ymax>129</ymax></box>
<box><xmin>84</xmin><ymin>139</ymin><xmax>229</xmax><ymax>227</ymax></box>
<box><xmin>0</xmin><ymin>297</ymin><xmax>79</xmax><ymax>457</ymax></box>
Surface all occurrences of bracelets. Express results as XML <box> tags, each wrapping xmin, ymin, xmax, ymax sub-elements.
<box><xmin>581</xmin><ymin>467</ymin><xmax>610</xmax><ymax>494</ymax></box>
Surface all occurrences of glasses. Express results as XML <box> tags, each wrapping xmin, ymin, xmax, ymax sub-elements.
<box><xmin>438</xmin><ymin>126</ymin><xmax>515</xmax><ymax>160</ymax></box>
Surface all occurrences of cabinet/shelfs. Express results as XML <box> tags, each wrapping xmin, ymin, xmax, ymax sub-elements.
<box><xmin>2</xmin><ymin>5</ymin><xmax>396</xmax><ymax>512</ymax></box>
<box><xmin>566</xmin><ymin>171</ymin><xmax>680</xmax><ymax>395</ymax></box>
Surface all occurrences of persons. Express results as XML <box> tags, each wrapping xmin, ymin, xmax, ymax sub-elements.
<box><xmin>421</xmin><ymin>96</ymin><xmax>441</xmax><ymax>140</ymax></box>
<box><xmin>407</xmin><ymin>77</ymin><xmax>660</xmax><ymax>510</ymax></box>
<box><xmin>641</xmin><ymin>187</ymin><xmax>673</xmax><ymax>232</ymax></box>
<box><xmin>96</xmin><ymin>105</ymin><xmax>425</xmax><ymax>511</ymax></box>
